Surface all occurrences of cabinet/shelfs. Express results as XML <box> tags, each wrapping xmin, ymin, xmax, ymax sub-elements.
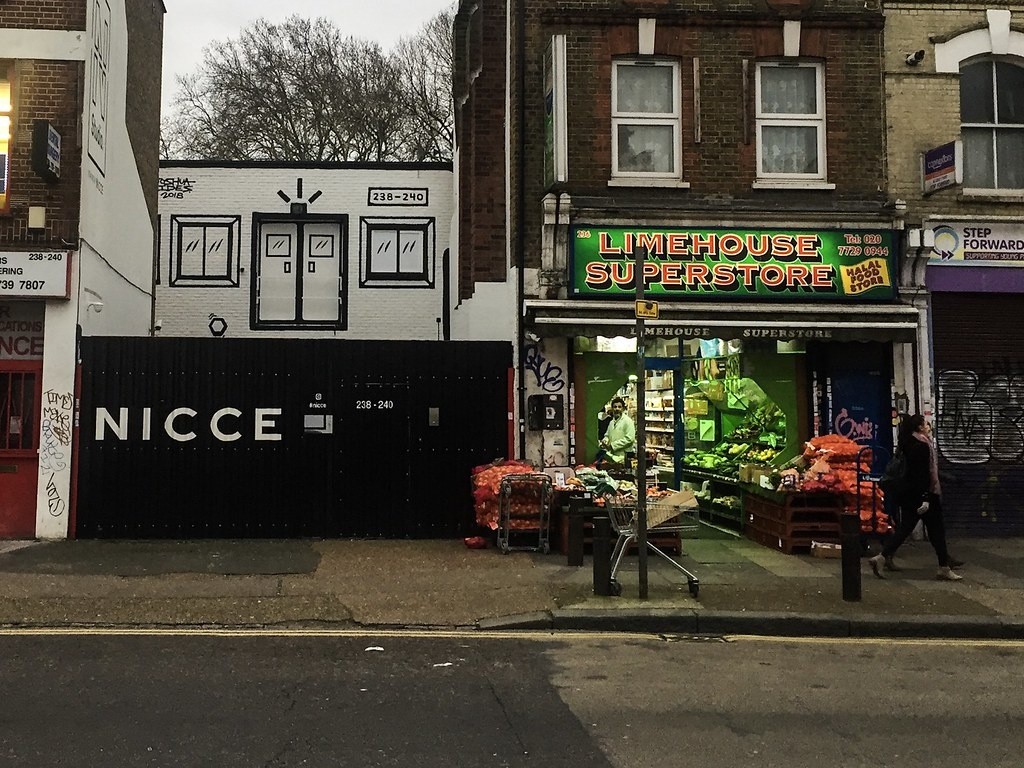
<box><xmin>681</xmin><ymin>353</ymin><xmax>800</xmax><ymax>537</ymax></box>
<box><xmin>605</xmin><ymin>370</ymin><xmax>674</xmax><ymax>474</ymax></box>
<box><xmin>739</xmin><ymin>482</ymin><xmax>852</xmax><ymax>555</ymax></box>
<box><xmin>561</xmin><ymin>506</ymin><xmax>685</xmax><ymax>558</ymax></box>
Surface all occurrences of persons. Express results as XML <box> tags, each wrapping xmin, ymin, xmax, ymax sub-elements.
<box><xmin>869</xmin><ymin>414</ymin><xmax>964</xmax><ymax>581</ymax></box>
<box><xmin>597</xmin><ymin>404</ymin><xmax>612</xmax><ymax>447</ymax></box>
<box><xmin>601</xmin><ymin>397</ymin><xmax>635</xmax><ymax>467</ymax></box>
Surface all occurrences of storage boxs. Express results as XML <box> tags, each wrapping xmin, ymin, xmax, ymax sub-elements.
<box><xmin>810</xmin><ymin>541</ymin><xmax>842</xmax><ymax>559</ymax></box>
<box><xmin>739</xmin><ymin>463</ymin><xmax>782</xmax><ymax>485</ymax></box>
<box><xmin>779</xmin><ymin>455</ymin><xmax>808</xmax><ymax>473</ymax></box>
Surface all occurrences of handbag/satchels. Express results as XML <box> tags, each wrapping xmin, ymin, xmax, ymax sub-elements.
<box><xmin>879</xmin><ymin>450</ymin><xmax>905</xmax><ymax>497</ymax></box>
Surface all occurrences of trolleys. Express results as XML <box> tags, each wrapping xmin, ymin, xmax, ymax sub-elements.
<box><xmin>591</xmin><ymin>472</ymin><xmax>700</xmax><ymax>597</ymax></box>
<box><xmin>497</xmin><ymin>472</ymin><xmax>554</xmax><ymax>556</ymax></box>
<box><xmin>856</xmin><ymin>446</ymin><xmax>897</xmax><ymax>558</ymax></box>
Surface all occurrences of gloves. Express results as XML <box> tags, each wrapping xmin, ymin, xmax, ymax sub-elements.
<box><xmin>917</xmin><ymin>501</ymin><xmax>930</xmax><ymax>515</ymax></box>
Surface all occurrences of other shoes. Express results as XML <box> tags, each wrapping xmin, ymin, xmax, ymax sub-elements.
<box><xmin>937</xmin><ymin>567</ymin><xmax>963</xmax><ymax>581</ymax></box>
<box><xmin>887</xmin><ymin>553</ymin><xmax>901</xmax><ymax>571</ymax></box>
<box><xmin>870</xmin><ymin>554</ymin><xmax>888</xmax><ymax>580</ymax></box>
<box><xmin>946</xmin><ymin>556</ymin><xmax>965</xmax><ymax>567</ymax></box>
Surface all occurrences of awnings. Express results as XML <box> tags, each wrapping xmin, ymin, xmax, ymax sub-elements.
<box><xmin>521</xmin><ymin>299</ymin><xmax>919</xmax><ymax>344</ymax></box>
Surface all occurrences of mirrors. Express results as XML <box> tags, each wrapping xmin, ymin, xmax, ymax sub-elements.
<box><xmin>695</xmin><ymin>377</ymin><xmax>786</xmax><ymax>434</ymax></box>
